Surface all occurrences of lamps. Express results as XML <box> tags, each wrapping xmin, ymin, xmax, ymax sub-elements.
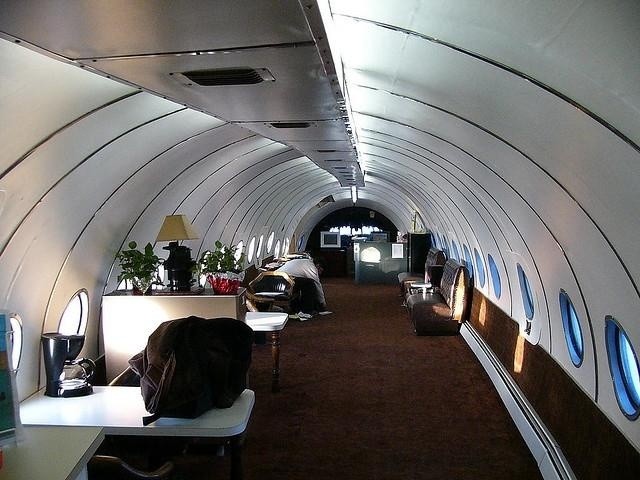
<box><xmin>155</xmin><ymin>214</ymin><xmax>202</xmax><ymax>291</ymax></box>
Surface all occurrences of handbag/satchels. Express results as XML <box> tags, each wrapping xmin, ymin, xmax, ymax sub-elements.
<box><xmin>128</xmin><ymin>317</ymin><xmax>255</xmax><ymax>426</ymax></box>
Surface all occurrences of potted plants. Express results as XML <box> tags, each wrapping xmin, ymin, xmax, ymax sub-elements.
<box><xmin>189</xmin><ymin>240</ymin><xmax>249</xmax><ymax>295</ymax></box>
<box><xmin>112</xmin><ymin>240</ymin><xmax>160</xmax><ymax>294</ymax></box>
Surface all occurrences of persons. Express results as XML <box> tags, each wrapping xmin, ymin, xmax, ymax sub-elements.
<box><xmin>274</xmin><ymin>256</ymin><xmax>333</xmax><ymax>320</ymax></box>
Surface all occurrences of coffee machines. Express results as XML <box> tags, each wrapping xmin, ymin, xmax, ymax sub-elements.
<box><xmin>41</xmin><ymin>331</ymin><xmax>96</xmax><ymax>397</ymax></box>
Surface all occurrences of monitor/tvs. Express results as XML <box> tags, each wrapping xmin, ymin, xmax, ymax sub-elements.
<box><xmin>321</xmin><ymin>232</ymin><xmax>341</xmax><ymax>248</ymax></box>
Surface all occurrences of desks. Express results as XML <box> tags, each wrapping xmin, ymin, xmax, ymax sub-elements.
<box><xmin>243</xmin><ymin>311</ymin><xmax>290</xmax><ymax>394</ymax></box>
<box><xmin>20</xmin><ymin>386</ymin><xmax>257</xmax><ymax>480</ymax></box>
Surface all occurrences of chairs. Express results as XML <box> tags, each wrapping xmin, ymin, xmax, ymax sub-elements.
<box><xmin>244</xmin><ymin>271</ymin><xmax>295</xmax><ymax>312</ymax></box>
<box><xmin>398</xmin><ymin>246</ymin><xmax>470</xmax><ymax>335</ymax></box>
